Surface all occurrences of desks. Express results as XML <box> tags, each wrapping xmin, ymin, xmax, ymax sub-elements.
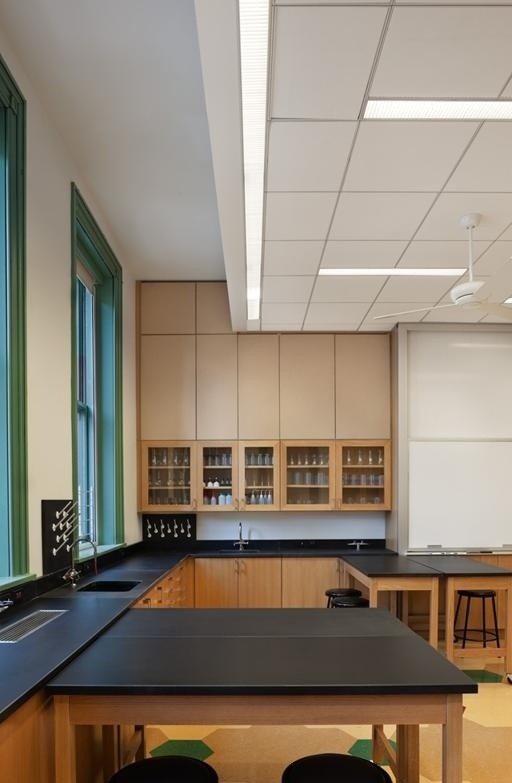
<box><xmin>333</xmin><ymin>553</ymin><xmax>444</xmax><ymax>658</ymax></box>
<box><xmin>408</xmin><ymin>550</ymin><xmax>512</xmax><ymax>689</ymax></box>
<box><xmin>36</xmin><ymin>637</ymin><xmax>483</xmax><ymax>782</ymax></box>
<box><xmin>98</xmin><ymin>600</ymin><xmax>415</xmax><ymax>783</ymax></box>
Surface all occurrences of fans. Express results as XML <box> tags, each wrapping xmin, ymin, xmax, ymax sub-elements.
<box><xmin>371</xmin><ymin>208</ymin><xmax>512</xmax><ymax>325</ymax></box>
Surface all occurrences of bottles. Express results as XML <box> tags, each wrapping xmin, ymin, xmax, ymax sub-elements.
<box><xmin>147</xmin><ymin>490</ymin><xmax>384</xmax><ymax>504</ymax></box>
<box><xmin>148</xmin><ymin>470</ymin><xmax>382</xmax><ymax>488</ymax></box>
<box><xmin>150</xmin><ymin>447</ymin><xmax>382</xmax><ymax>464</ymax></box>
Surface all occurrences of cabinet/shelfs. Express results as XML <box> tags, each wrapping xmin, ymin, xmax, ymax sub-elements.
<box><xmin>398</xmin><ymin>572</ymin><xmax>456</xmax><ymax>642</ymax></box>
<box><xmin>0</xmin><ymin>689</ymin><xmax>61</xmax><ymax>782</ymax></box>
<box><xmin>137</xmin><ymin>437</ymin><xmax>394</xmax><ymax>514</ymax></box>
<box><xmin>73</xmin><ymin>703</ymin><xmax>148</xmax><ymax>780</ymax></box>
<box><xmin>449</xmin><ymin>553</ymin><xmax>512</xmax><ymax>633</ymax></box>
<box><xmin>132</xmin><ymin>555</ymin><xmax>394</xmax><ymax>614</ymax></box>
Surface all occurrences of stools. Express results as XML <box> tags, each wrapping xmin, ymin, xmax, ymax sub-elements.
<box><xmin>450</xmin><ymin>589</ymin><xmax>503</xmax><ymax>652</ymax></box>
<box><xmin>279</xmin><ymin>750</ymin><xmax>393</xmax><ymax>781</ymax></box>
<box><xmin>105</xmin><ymin>753</ymin><xmax>221</xmax><ymax>782</ymax></box>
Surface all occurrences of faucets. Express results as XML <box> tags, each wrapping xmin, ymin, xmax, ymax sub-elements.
<box><xmin>238</xmin><ymin>522</ymin><xmax>244</xmax><ymax>550</ymax></box>
<box><xmin>63</xmin><ymin>539</ymin><xmax>98</xmax><ymax>585</ymax></box>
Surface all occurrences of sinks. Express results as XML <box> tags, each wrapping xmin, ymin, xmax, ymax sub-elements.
<box><xmin>77</xmin><ymin>579</ymin><xmax>149</xmax><ymax>598</ymax></box>
<box><xmin>218</xmin><ymin>549</ymin><xmax>261</xmax><ymax>557</ymax></box>
